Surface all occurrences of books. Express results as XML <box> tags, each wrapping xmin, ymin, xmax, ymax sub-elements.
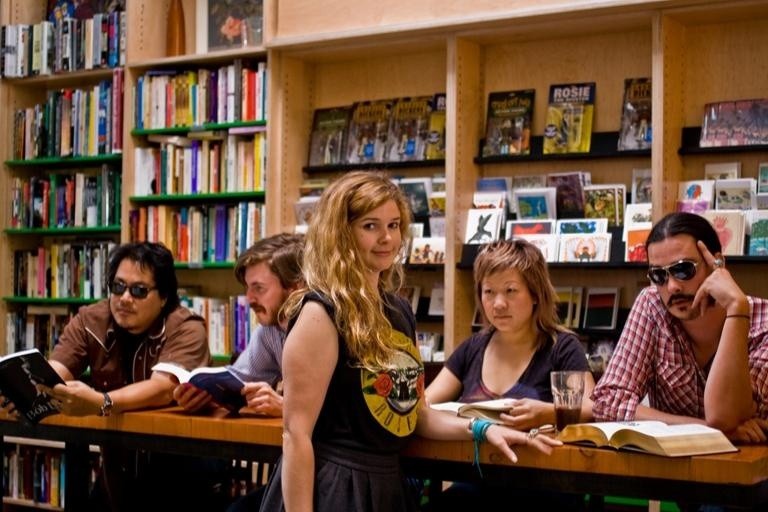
<box><xmin>429</xmin><ymin>399</ymin><xmax>554</xmax><ymax>430</ymax></box>
<box><xmin>307</xmin><ymin>93</ymin><xmax>446</xmax><ymax>168</ymax></box>
<box><xmin>675</xmin><ymin>162</ymin><xmax>767</xmax><ymax>256</ymax></box>
<box><xmin>554</xmin><ymin>421</ymin><xmax>740</xmax><ymax>458</ymax></box>
<box><xmin>294</xmin><ymin>172</ymin><xmax>445</xmax><ymax>363</ymax></box>
<box><xmin>701</xmin><ymin>99</ymin><xmax>767</xmax><ymax>147</ymax></box>
<box><xmin>481</xmin><ymin>78</ymin><xmax>652</xmax><ymax>157</ymax></box>
<box><xmin>463</xmin><ymin>168</ymin><xmax>652</xmax><ymax>373</ymax></box>
<box><xmin>0</xmin><ymin>0</ymin><xmax>266</xmax><ymax>512</ymax></box>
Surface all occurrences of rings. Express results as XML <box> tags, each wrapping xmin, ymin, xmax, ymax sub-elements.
<box><xmin>529</xmin><ymin>427</ymin><xmax>540</xmax><ymax>438</ymax></box>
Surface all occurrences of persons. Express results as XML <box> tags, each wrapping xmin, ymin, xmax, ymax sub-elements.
<box><xmin>171</xmin><ymin>231</ymin><xmax>311</xmax><ymax>512</ymax></box>
<box><xmin>257</xmin><ymin>170</ymin><xmax>564</xmax><ymax>512</ymax></box>
<box><xmin>425</xmin><ymin>238</ymin><xmax>596</xmax><ymax>512</ymax></box>
<box><xmin>588</xmin><ymin>211</ymin><xmax>768</xmax><ymax>512</ymax></box>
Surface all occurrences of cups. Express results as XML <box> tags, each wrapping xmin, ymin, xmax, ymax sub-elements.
<box><xmin>551</xmin><ymin>370</ymin><xmax>584</xmax><ymax>428</ymax></box>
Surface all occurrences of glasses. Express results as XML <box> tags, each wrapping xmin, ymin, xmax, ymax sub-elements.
<box><xmin>646</xmin><ymin>260</ymin><xmax>704</xmax><ymax>287</ymax></box>
<box><xmin>106</xmin><ymin>279</ymin><xmax>158</xmax><ymax>298</ymax></box>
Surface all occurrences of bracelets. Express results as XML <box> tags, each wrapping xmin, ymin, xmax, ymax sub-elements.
<box><xmin>471</xmin><ymin>419</ymin><xmax>495</xmax><ymax>480</ymax></box>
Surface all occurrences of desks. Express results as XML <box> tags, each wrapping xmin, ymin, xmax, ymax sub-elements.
<box><xmin>2</xmin><ymin>376</ymin><xmax>766</xmax><ymax>511</ymax></box>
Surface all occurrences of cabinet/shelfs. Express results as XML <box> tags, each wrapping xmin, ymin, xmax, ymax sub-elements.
<box><xmin>124</xmin><ymin>0</ymin><xmax>264</xmax><ymax>369</ymax></box>
<box><xmin>0</xmin><ymin>0</ymin><xmax>124</xmax><ymax>510</ymax></box>
<box><xmin>274</xmin><ymin>23</ymin><xmax>445</xmax><ymax>359</ymax></box>
<box><xmin>662</xmin><ymin>0</ymin><xmax>768</xmax><ymax>296</ymax></box>
<box><xmin>453</xmin><ymin>1</ymin><xmax>658</xmax><ymax>367</ymax></box>
